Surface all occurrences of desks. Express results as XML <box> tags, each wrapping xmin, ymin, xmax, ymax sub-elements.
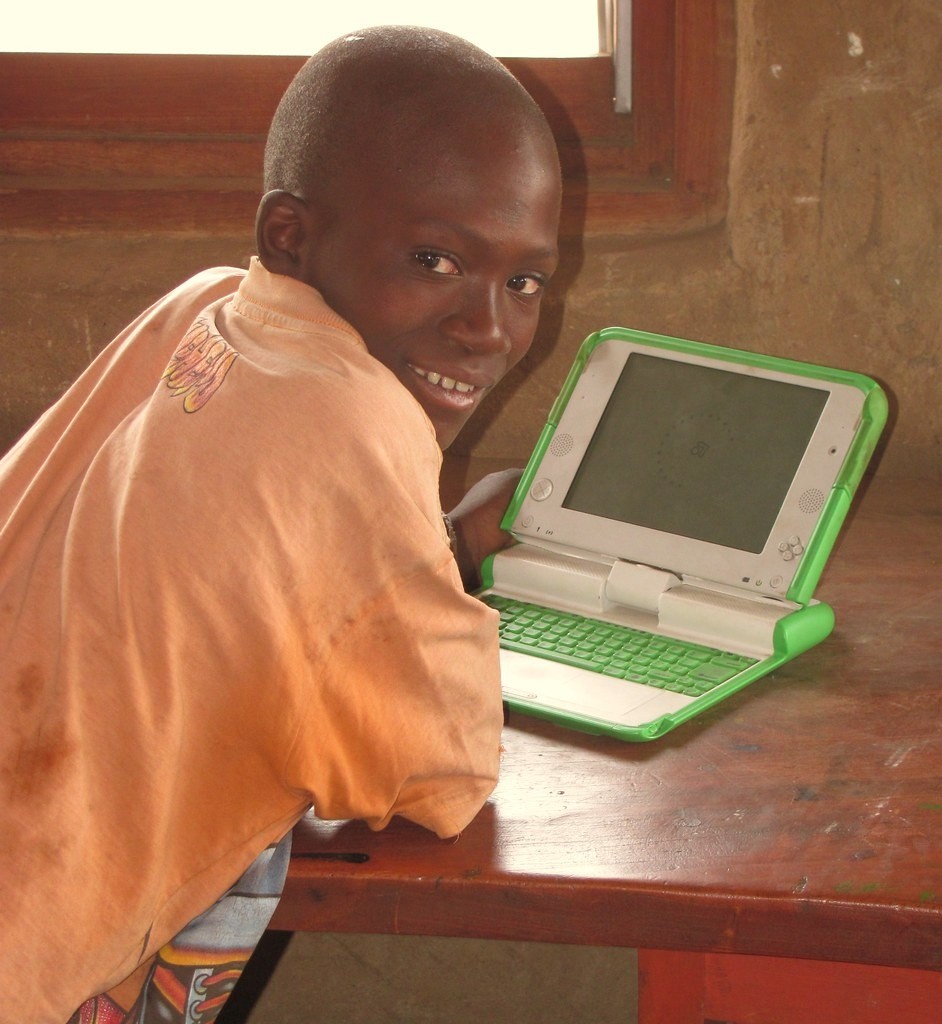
<box><xmin>266</xmin><ymin>456</ymin><xmax>942</xmax><ymax>1022</ymax></box>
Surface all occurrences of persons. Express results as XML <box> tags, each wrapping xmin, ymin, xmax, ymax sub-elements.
<box><xmin>0</xmin><ymin>24</ymin><xmax>566</xmax><ymax>1024</ymax></box>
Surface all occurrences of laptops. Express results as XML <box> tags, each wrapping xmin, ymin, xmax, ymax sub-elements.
<box><xmin>468</xmin><ymin>326</ymin><xmax>888</xmax><ymax>741</ymax></box>
